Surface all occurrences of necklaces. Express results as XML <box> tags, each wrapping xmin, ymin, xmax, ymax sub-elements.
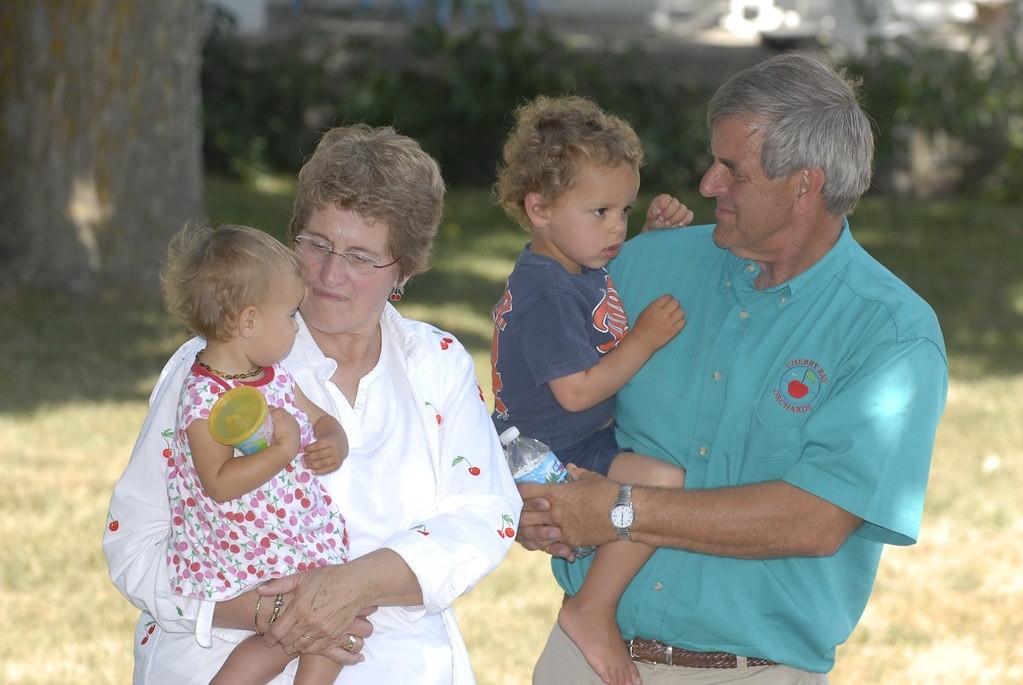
<box><xmin>194</xmin><ymin>349</ymin><xmax>263</xmax><ymax>380</ymax></box>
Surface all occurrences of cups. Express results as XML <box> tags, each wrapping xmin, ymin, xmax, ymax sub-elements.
<box><xmin>208</xmin><ymin>386</ymin><xmax>275</xmax><ymax>456</ymax></box>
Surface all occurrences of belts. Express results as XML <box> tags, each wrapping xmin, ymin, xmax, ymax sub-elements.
<box><xmin>623</xmin><ymin>637</ymin><xmax>779</xmax><ymax>668</ymax></box>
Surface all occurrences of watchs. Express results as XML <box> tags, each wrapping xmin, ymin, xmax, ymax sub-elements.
<box><xmin>609</xmin><ymin>483</ymin><xmax>635</xmax><ymax>541</ymax></box>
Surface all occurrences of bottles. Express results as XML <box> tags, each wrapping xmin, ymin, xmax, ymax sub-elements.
<box><xmin>499</xmin><ymin>426</ymin><xmax>596</xmax><ymax>559</ymax></box>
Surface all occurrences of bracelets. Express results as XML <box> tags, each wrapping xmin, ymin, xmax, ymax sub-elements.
<box><xmin>255</xmin><ymin>595</ymin><xmax>261</xmax><ymax>636</ymax></box>
<box><xmin>268</xmin><ymin>594</ymin><xmax>283</xmax><ymax>626</ymax></box>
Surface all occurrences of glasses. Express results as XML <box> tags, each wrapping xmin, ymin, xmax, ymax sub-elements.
<box><xmin>290</xmin><ymin>217</ymin><xmax>404</xmax><ymax>276</ymax></box>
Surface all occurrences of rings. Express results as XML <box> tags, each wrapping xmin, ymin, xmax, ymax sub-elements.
<box><xmin>303</xmin><ymin>635</ymin><xmax>315</xmax><ymax>642</ymax></box>
<box><xmin>343</xmin><ymin>635</ymin><xmax>355</xmax><ymax>650</ymax></box>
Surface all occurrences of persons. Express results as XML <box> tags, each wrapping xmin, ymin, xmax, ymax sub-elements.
<box><xmin>516</xmin><ymin>57</ymin><xmax>949</xmax><ymax>685</ymax></box>
<box><xmin>496</xmin><ymin>96</ymin><xmax>695</xmax><ymax>684</ymax></box>
<box><xmin>164</xmin><ymin>226</ymin><xmax>349</xmax><ymax>685</ymax></box>
<box><xmin>102</xmin><ymin>124</ymin><xmax>523</xmax><ymax>685</ymax></box>
